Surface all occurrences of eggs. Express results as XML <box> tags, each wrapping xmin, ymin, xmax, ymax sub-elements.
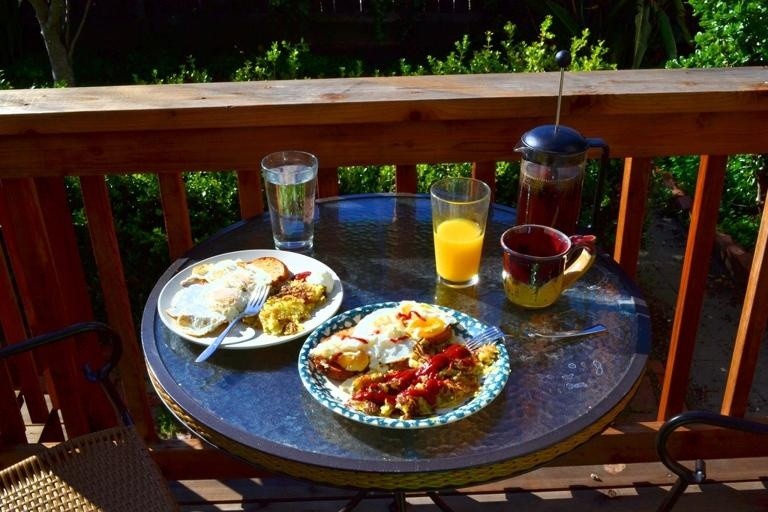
<box><xmin>161</xmin><ymin>259</ymin><xmax>261</xmax><ymax>338</ymax></box>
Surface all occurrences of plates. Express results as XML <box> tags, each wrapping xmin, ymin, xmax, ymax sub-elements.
<box><xmin>157</xmin><ymin>249</ymin><xmax>343</xmax><ymax>348</ymax></box>
<box><xmin>299</xmin><ymin>301</ymin><xmax>511</xmax><ymax>428</ymax></box>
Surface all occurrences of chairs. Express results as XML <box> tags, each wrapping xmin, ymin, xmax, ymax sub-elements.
<box><xmin>657</xmin><ymin>411</ymin><xmax>767</xmax><ymax>510</ymax></box>
<box><xmin>0</xmin><ymin>322</ymin><xmax>180</xmax><ymax>511</ymax></box>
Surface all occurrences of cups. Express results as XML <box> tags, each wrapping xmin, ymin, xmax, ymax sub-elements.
<box><xmin>261</xmin><ymin>151</ymin><xmax>319</xmax><ymax>248</ymax></box>
<box><xmin>500</xmin><ymin>224</ymin><xmax>597</xmax><ymax>309</ymax></box>
<box><xmin>430</xmin><ymin>177</ymin><xmax>492</xmax><ymax>288</ymax></box>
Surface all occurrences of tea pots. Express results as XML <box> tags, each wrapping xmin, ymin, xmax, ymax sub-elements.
<box><xmin>513</xmin><ymin>125</ymin><xmax>611</xmax><ymax>234</ymax></box>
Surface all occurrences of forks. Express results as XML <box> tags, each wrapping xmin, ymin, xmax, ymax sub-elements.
<box><xmin>467</xmin><ymin>325</ymin><xmax>607</xmax><ymax>350</ymax></box>
<box><xmin>195</xmin><ymin>285</ymin><xmax>272</xmax><ymax>363</ymax></box>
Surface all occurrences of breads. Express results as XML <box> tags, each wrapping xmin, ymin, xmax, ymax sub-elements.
<box><xmin>242</xmin><ymin>256</ymin><xmax>289</xmax><ymax>295</ymax></box>
